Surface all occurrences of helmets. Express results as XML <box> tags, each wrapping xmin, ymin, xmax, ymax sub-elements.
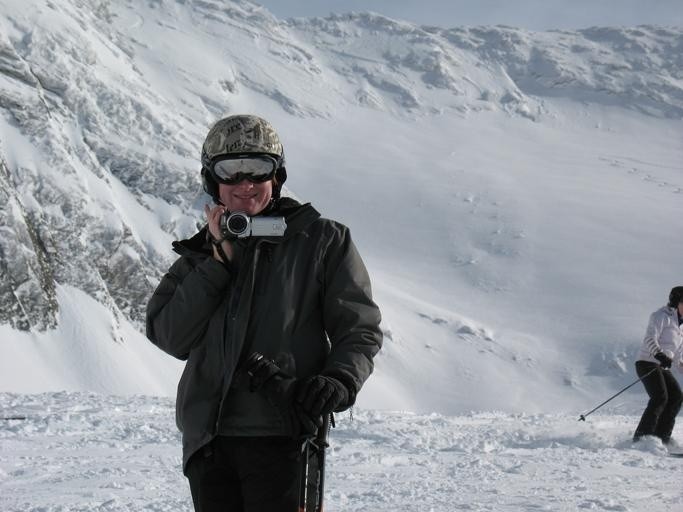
<box><xmin>199</xmin><ymin>114</ymin><xmax>286</xmax><ymax>195</ymax></box>
<box><xmin>669</xmin><ymin>286</ymin><xmax>683</xmax><ymax>308</ymax></box>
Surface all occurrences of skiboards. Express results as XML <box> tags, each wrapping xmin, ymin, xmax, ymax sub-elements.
<box><xmin>630</xmin><ymin>440</ymin><xmax>683</xmax><ymax>458</ymax></box>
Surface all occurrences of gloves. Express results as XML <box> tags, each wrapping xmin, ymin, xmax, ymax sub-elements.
<box><xmin>240</xmin><ymin>352</ymin><xmax>357</xmax><ymax>432</ymax></box>
<box><xmin>653</xmin><ymin>352</ymin><xmax>672</xmax><ymax>369</ymax></box>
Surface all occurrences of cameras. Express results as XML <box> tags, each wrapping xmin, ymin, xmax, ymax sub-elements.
<box><xmin>218</xmin><ymin>210</ymin><xmax>288</xmax><ymax>240</ymax></box>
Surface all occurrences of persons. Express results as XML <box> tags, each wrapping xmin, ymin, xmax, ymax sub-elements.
<box><xmin>633</xmin><ymin>287</ymin><xmax>683</xmax><ymax>454</ymax></box>
<box><xmin>146</xmin><ymin>115</ymin><xmax>382</xmax><ymax>512</ymax></box>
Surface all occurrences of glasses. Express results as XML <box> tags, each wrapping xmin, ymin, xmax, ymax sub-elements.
<box><xmin>213</xmin><ymin>156</ymin><xmax>276</xmax><ymax>181</ymax></box>
